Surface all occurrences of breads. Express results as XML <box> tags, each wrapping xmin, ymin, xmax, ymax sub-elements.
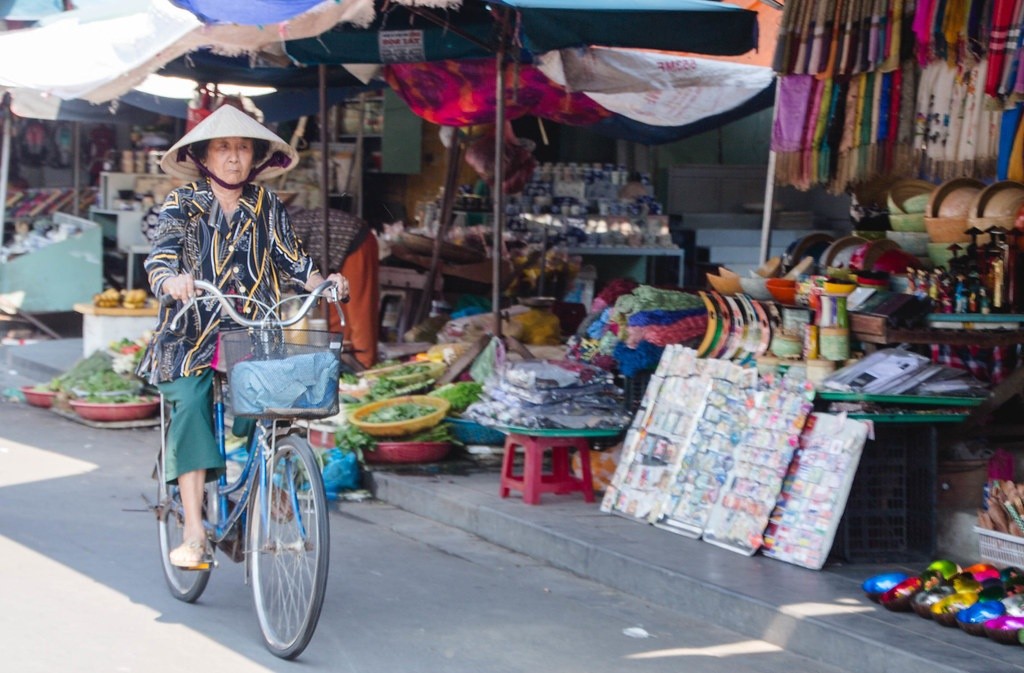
<box><xmin>95</xmin><ymin>287</ymin><xmax>147</xmax><ymax>310</ymax></box>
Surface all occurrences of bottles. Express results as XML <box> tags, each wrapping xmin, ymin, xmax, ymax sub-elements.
<box><xmin>502</xmin><ymin>161</ymin><xmax>670</xmax><ymax>247</ymax></box>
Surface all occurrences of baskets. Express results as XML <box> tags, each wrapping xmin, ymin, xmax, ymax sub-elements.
<box><xmin>68</xmin><ymin>396</ymin><xmax>160</xmax><ymax>421</ymax></box>
<box><xmin>347</xmin><ymin>396</ymin><xmax>450</xmax><ymax>437</ymax></box>
<box><xmin>385</xmin><ymin>361</ymin><xmax>446</xmax><ymax>385</ymax></box>
<box><xmin>360</xmin><ymin>441</ymin><xmax>451</xmax><ymax>465</ymax></box>
<box><xmin>218</xmin><ymin>329</ymin><xmax>343</xmax><ymax>421</ymax></box>
<box><xmin>444</xmin><ymin>419</ymin><xmax>505</xmax><ymax>446</ymax></box>
<box><xmin>860</xmin><ymin>568</ymin><xmax>1024</xmax><ymax>645</ymax></box>
<box><xmin>18</xmin><ymin>385</ymin><xmax>59</xmax><ymax>407</ymax></box>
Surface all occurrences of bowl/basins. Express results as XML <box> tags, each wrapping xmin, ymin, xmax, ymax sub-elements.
<box><xmin>922</xmin><ymin>218</ymin><xmax>971</xmax><ymax>242</ymax></box>
<box><xmin>18</xmin><ymin>386</ymin><xmax>73</xmax><ymax>407</ymax></box>
<box><xmin>921</xmin><ymin>243</ymin><xmax>970</xmax><ymax>271</ymax></box>
<box><xmin>968</xmin><ymin>216</ymin><xmax>1013</xmax><ymax>244</ymax></box>
<box><xmin>445</xmin><ymin>418</ymin><xmax>508</xmax><ymax>445</ymax></box>
<box><xmin>347</xmin><ymin>396</ymin><xmax>448</xmax><ymax>438</ymax></box>
<box><xmin>926</xmin><ymin>176</ymin><xmax>985</xmax><ymax>218</ymax></box>
<box><xmin>887</xmin><ymin>180</ymin><xmax>933</xmax><ymax>213</ymax></box>
<box><xmin>707</xmin><ymin>274</ymin><xmax>740</xmax><ymax>295</ymax></box>
<box><xmin>767</xmin><ymin>280</ymin><xmax>797</xmax><ymax>303</ymax></box>
<box><xmin>884</xmin><ymin>231</ymin><xmax>928</xmax><ymax>256</ymax></box>
<box><xmin>365</xmin><ymin>440</ymin><xmax>447</xmax><ymax>464</ymax></box>
<box><xmin>69</xmin><ymin>398</ymin><xmax>161</xmax><ymax>419</ymax></box>
<box><xmin>971</xmin><ymin>181</ymin><xmax>1024</xmax><ymax>218</ymax></box>
<box><xmin>718</xmin><ymin>267</ymin><xmax>738</xmax><ymax>277</ymax></box>
<box><xmin>890</xmin><ymin>215</ymin><xmax>928</xmax><ymax>231</ymax></box>
<box><xmin>739</xmin><ymin>278</ymin><xmax>769</xmax><ymax>299</ymax></box>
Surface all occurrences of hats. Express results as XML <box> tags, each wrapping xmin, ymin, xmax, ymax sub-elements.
<box><xmin>160</xmin><ymin>103</ymin><xmax>299</xmax><ymax>181</ymax></box>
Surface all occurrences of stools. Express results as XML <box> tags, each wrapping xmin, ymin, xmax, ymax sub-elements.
<box><xmin>500</xmin><ymin>434</ymin><xmax>596</xmax><ymax>505</ymax></box>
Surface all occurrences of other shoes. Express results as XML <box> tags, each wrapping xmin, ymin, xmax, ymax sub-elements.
<box><xmin>271</xmin><ymin>488</ymin><xmax>294</xmax><ymax>524</ymax></box>
<box><xmin>170</xmin><ymin>541</ymin><xmax>212</xmax><ymax>567</ymax></box>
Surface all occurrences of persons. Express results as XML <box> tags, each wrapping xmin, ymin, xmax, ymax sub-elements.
<box><xmin>143</xmin><ymin>104</ymin><xmax>350</xmax><ymax>571</ymax></box>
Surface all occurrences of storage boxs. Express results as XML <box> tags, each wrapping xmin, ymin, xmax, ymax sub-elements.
<box><xmin>835</xmin><ymin>440</ymin><xmax>906</xmax><ymax>564</ymax></box>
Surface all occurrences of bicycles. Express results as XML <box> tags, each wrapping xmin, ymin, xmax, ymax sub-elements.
<box><xmin>147</xmin><ymin>281</ymin><xmax>351</xmax><ymax>660</ymax></box>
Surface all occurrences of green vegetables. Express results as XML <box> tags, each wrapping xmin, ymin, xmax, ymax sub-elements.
<box><xmin>44</xmin><ymin>349</ymin><xmax>159</xmax><ymax>406</ymax></box>
<box><xmin>332</xmin><ymin>358</ymin><xmax>482</xmax><ymax>452</ymax></box>
